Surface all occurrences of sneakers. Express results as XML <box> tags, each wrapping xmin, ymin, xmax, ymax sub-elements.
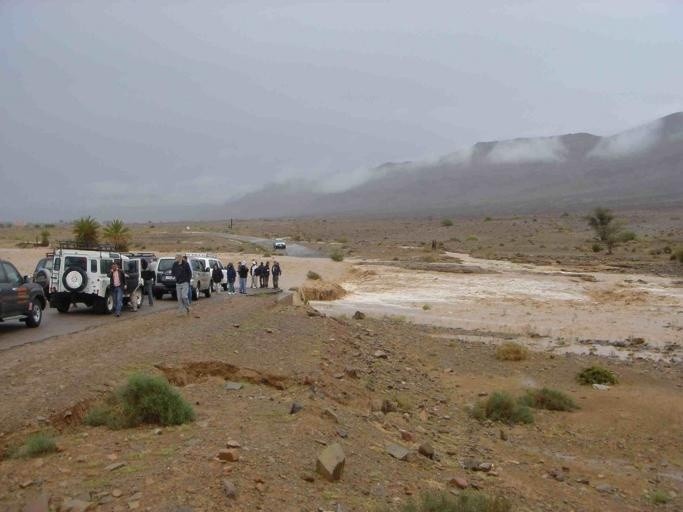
<box><xmin>215</xmin><ymin>286</ymin><xmax>278</xmax><ymax>295</ymax></box>
<box><xmin>111</xmin><ymin>301</ymin><xmax>192</xmax><ymax>317</ymax></box>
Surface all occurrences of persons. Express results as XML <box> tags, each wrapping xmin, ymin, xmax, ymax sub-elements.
<box><xmin>139</xmin><ymin>261</ymin><xmax>156</xmax><ymax>305</ymax></box>
<box><xmin>108</xmin><ymin>263</ymin><xmax>127</xmax><ymax>316</ymax></box>
<box><xmin>151</xmin><ymin>258</ymin><xmax>157</xmax><ymax>280</ymax></box>
<box><xmin>184</xmin><ymin>255</ymin><xmax>194</xmax><ymax>303</ymax></box>
<box><xmin>171</xmin><ymin>254</ymin><xmax>190</xmax><ymax>316</ymax></box>
<box><xmin>213</xmin><ymin>258</ymin><xmax>282</xmax><ymax>292</ymax></box>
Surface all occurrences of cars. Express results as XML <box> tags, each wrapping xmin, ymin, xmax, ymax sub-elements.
<box><xmin>272</xmin><ymin>238</ymin><xmax>286</xmax><ymax>249</ymax></box>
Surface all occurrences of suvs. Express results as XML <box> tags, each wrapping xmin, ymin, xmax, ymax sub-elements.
<box><xmin>198</xmin><ymin>255</ymin><xmax>229</xmax><ymax>291</ymax></box>
<box><xmin>30</xmin><ymin>251</ymin><xmax>53</xmax><ymax>291</ymax></box>
<box><xmin>46</xmin><ymin>238</ymin><xmax>145</xmax><ymax>314</ymax></box>
<box><xmin>150</xmin><ymin>253</ymin><xmax>214</xmax><ymax>302</ymax></box>
<box><xmin>121</xmin><ymin>249</ymin><xmax>158</xmax><ymax>297</ymax></box>
<box><xmin>0</xmin><ymin>257</ymin><xmax>50</xmax><ymax>330</ymax></box>
